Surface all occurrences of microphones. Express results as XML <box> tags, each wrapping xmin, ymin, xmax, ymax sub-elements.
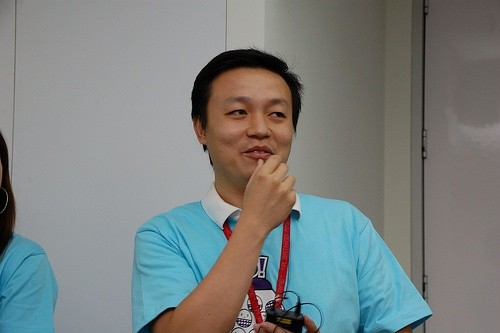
<box><xmin>265</xmin><ymin>288</ymin><xmax>305</xmax><ymax>333</ymax></box>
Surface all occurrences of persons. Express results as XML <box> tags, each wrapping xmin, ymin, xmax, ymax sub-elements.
<box><xmin>130</xmin><ymin>48</ymin><xmax>435</xmax><ymax>333</ymax></box>
<box><xmin>0</xmin><ymin>129</ymin><xmax>60</xmax><ymax>333</ymax></box>
